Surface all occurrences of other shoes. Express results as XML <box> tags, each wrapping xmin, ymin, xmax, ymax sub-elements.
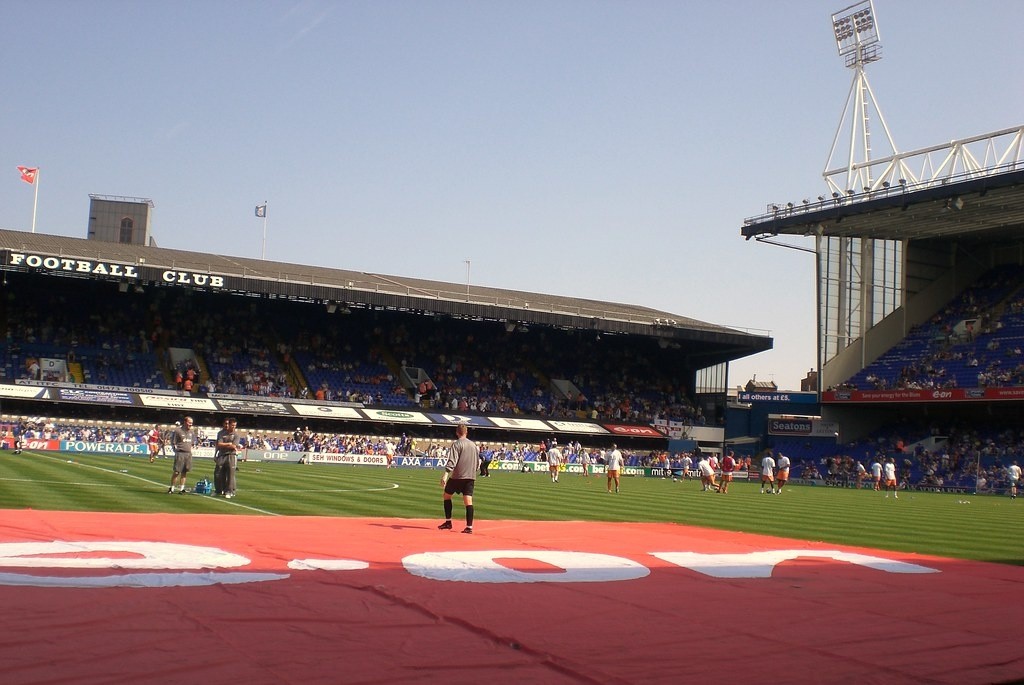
<box><xmin>230</xmin><ymin>491</ymin><xmax>236</xmax><ymax>497</ymax></box>
<box><xmin>723</xmin><ymin>490</ymin><xmax>728</xmax><ymax>493</ymax></box>
<box><xmin>873</xmin><ymin>488</ymin><xmax>879</xmax><ymax>491</ymax></box>
<box><xmin>461</xmin><ymin>527</ymin><xmax>473</xmax><ymax>534</ymax></box>
<box><xmin>225</xmin><ymin>491</ymin><xmax>231</xmax><ymax>499</ymax></box>
<box><xmin>772</xmin><ymin>491</ymin><xmax>775</xmax><ymax>494</ymax></box>
<box><xmin>555</xmin><ymin>478</ymin><xmax>559</xmax><ymax>483</ymax></box>
<box><xmin>438</xmin><ymin>522</ymin><xmax>453</xmax><ymax>529</ymax></box>
<box><xmin>885</xmin><ymin>495</ymin><xmax>888</xmax><ymax>497</ymax></box>
<box><xmin>616</xmin><ymin>489</ymin><xmax>619</xmax><ymax>493</ymax></box>
<box><xmin>761</xmin><ymin>490</ymin><xmax>764</xmax><ymax>493</ymax></box>
<box><xmin>661</xmin><ymin>477</ymin><xmax>666</xmax><ymax>480</ymax></box>
<box><xmin>1010</xmin><ymin>496</ymin><xmax>1015</xmax><ymax>499</ymax></box>
<box><xmin>485</xmin><ymin>473</ymin><xmax>490</xmax><ymax>477</ymax></box>
<box><xmin>608</xmin><ymin>490</ymin><xmax>612</xmax><ymax>493</ymax></box>
<box><xmin>168</xmin><ymin>488</ymin><xmax>174</xmax><ymax>493</ymax></box>
<box><xmin>777</xmin><ymin>490</ymin><xmax>781</xmax><ymax>495</ymax></box>
<box><xmin>716</xmin><ymin>490</ymin><xmax>720</xmax><ymax>493</ymax></box>
<box><xmin>211</xmin><ymin>492</ymin><xmax>218</xmax><ymax>496</ymax></box>
<box><xmin>179</xmin><ymin>490</ymin><xmax>183</xmax><ymax>494</ymax></box>
<box><xmin>221</xmin><ymin>489</ymin><xmax>227</xmax><ymax>494</ymax></box>
<box><xmin>479</xmin><ymin>474</ymin><xmax>486</xmax><ymax>478</ymax></box>
<box><xmin>894</xmin><ymin>495</ymin><xmax>898</xmax><ymax>498</ymax></box>
<box><xmin>704</xmin><ymin>488</ymin><xmax>707</xmax><ymax>491</ymax></box>
<box><xmin>584</xmin><ymin>473</ymin><xmax>588</xmax><ymax>476</ymax></box>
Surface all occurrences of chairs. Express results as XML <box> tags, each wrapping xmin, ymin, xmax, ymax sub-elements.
<box><xmin>772</xmin><ymin>260</ymin><xmax>1024</xmax><ymax>488</ymax></box>
<box><xmin>1</xmin><ymin>273</ymin><xmax>700</xmax><ymax>468</ymax></box>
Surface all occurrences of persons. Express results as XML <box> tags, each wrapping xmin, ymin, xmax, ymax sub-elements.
<box><xmin>438</xmin><ymin>424</ymin><xmax>480</xmax><ymax>534</ymax></box>
<box><xmin>715</xmin><ymin>451</ymin><xmax>740</xmax><ymax>493</ymax></box>
<box><xmin>697</xmin><ymin>456</ymin><xmax>724</xmax><ymax>491</ymax></box>
<box><xmin>479</xmin><ymin>453</ymin><xmax>491</xmax><ymax>477</ymax></box>
<box><xmin>12</xmin><ymin>418</ymin><xmax>27</xmax><ymax>455</ymax></box>
<box><xmin>147</xmin><ymin>425</ymin><xmax>161</xmax><ymax>463</ymax></box>
<box><xmin>548</xmin><ymin>444</ymin><xmax>562</xmax><ymax>483</ymax></box>
<box><xmin>315</xmin><ymin>386</ymin><xmax>324</xmax><ymax>399</ymax></box>
<box><xmin>384</xmin><ymin>438</ymin><xmax>395</xmax><ymax>468</ymax></box>
<box><xmin>0</xmin><ymin>271</ymin><xmax>1024</xmax><ymax>501</ymax></box>
<box><xmin>680</xmin><ymin>453</ymin><xmax>693</xmax><ymax>482</ymax></box>
<box><xmin>176</xmin><ymin>369</ymin><xmax>183</xmax><ymax>389</ymax></box>
<box><xmin>211</xmin><ymin>418</ymin><xmax>240</xmax><ymax>498</ymax></box>
<box><xmin>661</xmin><ymin>456</ymin><xmax>669</xmax><ymax>478</ymax></box>
<box><xmin>766</xmin><ymin>451</ymin><xmax>790</xmax><ymax>494</ymax></box>
<box><xmin>581</xmin><ymin>449</ymin><xmax>590</xmax><ymax>476</ymax></box>
<box><xmin>168</xmin><ymin>417</ymin><xmax>194</xmax><ymax>493</ymax></box>
<box><xmin>155</xmin><ymin>428</ymin><xmax>168</xmax><ymax>458</ymax></box>
<box><xmin>761</xmin><ymin>451</ymin><xmax>776</xmax><ymax>494</ymax></box>
<box><xmin>603</xmin><ymin>444</ymin><xmax>624</xmax><ymax>494</ymax></box>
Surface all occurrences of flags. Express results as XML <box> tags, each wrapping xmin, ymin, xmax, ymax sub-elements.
<box><xmin>17</xmin><ymin>165</ymin><xmax>37</xmax><ymax>184</ymax></box>
